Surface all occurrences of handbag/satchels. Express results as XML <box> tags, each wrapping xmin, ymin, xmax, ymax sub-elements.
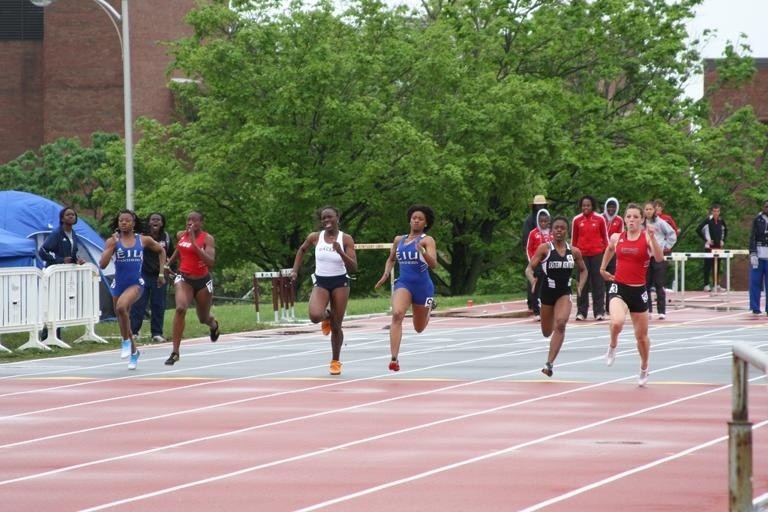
<box><xmin>158</xmin><ymin>273</ymin><xmax>164</xmax><ymax>278</ymax></box>
<box><xmin>420</xmin><ymin>247</ymin><xmax>426</xmax><ymax>256</ymax></box>
<box><xmin>163</xmin><ymin>264</ymin><xmax>170</xmax><ymax>270</ymax></box>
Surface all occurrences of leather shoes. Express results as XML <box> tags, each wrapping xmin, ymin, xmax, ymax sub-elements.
<box><xmin>750</xmin><ymin>252</ymin><xmax>759</xmax><ymax>266</ymax></box>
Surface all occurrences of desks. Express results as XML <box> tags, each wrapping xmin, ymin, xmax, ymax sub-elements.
<box><xmin>30</xmin><ymin>0</ymin><xmax>136</xmax><ymax>214</ymax></box>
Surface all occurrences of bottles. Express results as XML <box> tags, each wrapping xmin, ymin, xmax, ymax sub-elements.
<box><xmin>529</xmin><ymin>194</ymin><xmax>548</xmax><ymax>205</ymax></box>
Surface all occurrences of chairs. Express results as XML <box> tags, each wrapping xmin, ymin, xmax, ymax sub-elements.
<box><xmin>322</xmin><ymin>309</ymin><xmax>331</xmax><ymax>335</ymax></box>
<box><xmin>658</xmin><ymin>313</ymin><xmax>665</xmax><ymax>319</ymax></box>
<box><xmin>128</xmin><ymin>349</ymin><xmax>140</xmax><ymax>370</ymax></box>
<box><xmin>606</xmin><ymin>344</ymin><xmax>616</xmax><ymax>366</ymax></box>
<box><xmin>638</xmin><ymin>365</ymin><xmax>649</xmax><ymax>386</ymax></box>
<box><xmin>596</xmin><ymin>314</ymin><xmax>603</xmax><ymax>320</ymax></box>
<box><xmin>389</xmin><ymin>358</ymin><xmax>400</xmax><ymax>371</ymax></box>
<box><xmin>164</xmin><ymin>352</ymin><xmax>179</xmax><ymax>365</ymax></box>
<box><xmin>152</xmin><ymin>335</ymin><xmax>166</xmax><ymax>343</ymax></box>
<box><xmin>704</xmin><ymin>285</ymin><xmax>711</xmax><ymax>292</ymax></box>
<box><xmin>713</xmin><ymin>286</ymin><xmax>726</xmax><ymax>292</ymax></box>
<box><xmin>211</xmin><ymin>320</ymin><xmax>219</xmax><ymax>341</ymax></box>
<box><xmin>541</xmin><ymin>362</ymin><xmax>554</xmax><ymax>377</ymax></box>
<box><xmin>576</xmin><ymin>314</ymin><xmax>585</xmax><ymax>320</ymax></box>
<box><xmin>132</xmin><ymin>332</ymin><xmax>140</xmax><ymax>342</ymax></box>
<box><xmin>753</xmin><ymin>309</ymin><xmax>763</xmax><ymax>316</ymax></box>
<box><xmin>329</xmin><ymin>359</ymin><xmax>342</xmax><ymax>374</ymax></box>
<box><xmin>121</xmin><ymin>338</ymin><xmax>132</xmax><ymax>359</ymax></box>
<box><xmin>533</xmin><ymin>314</ymin><xmax>542</xmax><ymax>321</ymax></box>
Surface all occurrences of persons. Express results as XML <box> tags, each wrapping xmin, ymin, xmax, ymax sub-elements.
<box><xmin>372</xmin><ymin>205</ymin><xmax>441</xmax><ymax>372</ymax></box>
<box><xmin>287</xmin><ymin>207</ymin><xmax>359</xmax><ymax>377</ymax></box>
<box><xmin>521</xmin><ymin>193</ymin><xmax>767</xmax><ymax>322</ymax></box>
<box><xmin>600</xmin><ymin>203</ymin><xmax>665</xmax><ymax>386</ymax></box>
<box><xmin>129</xmin><ymin>212</ymin><xmax>176</xmax><ymax>343</ymax></box>
<box><xmin>161</xmin><ymin>211</ymin><xmax>220</xmax><ymax>366</ymax></box>
<box><xmin>525</xmin><ymin>215</ymin><xmax>588</xmax><ymax>378</ymax></box>
<box><xmin>100</xmin><ymin>209</ymin><xmax>167</xmax><ymax>370</ymax></box>
<box><xmin>41</xmin><ymin>207</ymin><xmax>87</xmax><ymax>347</ymax></box>
